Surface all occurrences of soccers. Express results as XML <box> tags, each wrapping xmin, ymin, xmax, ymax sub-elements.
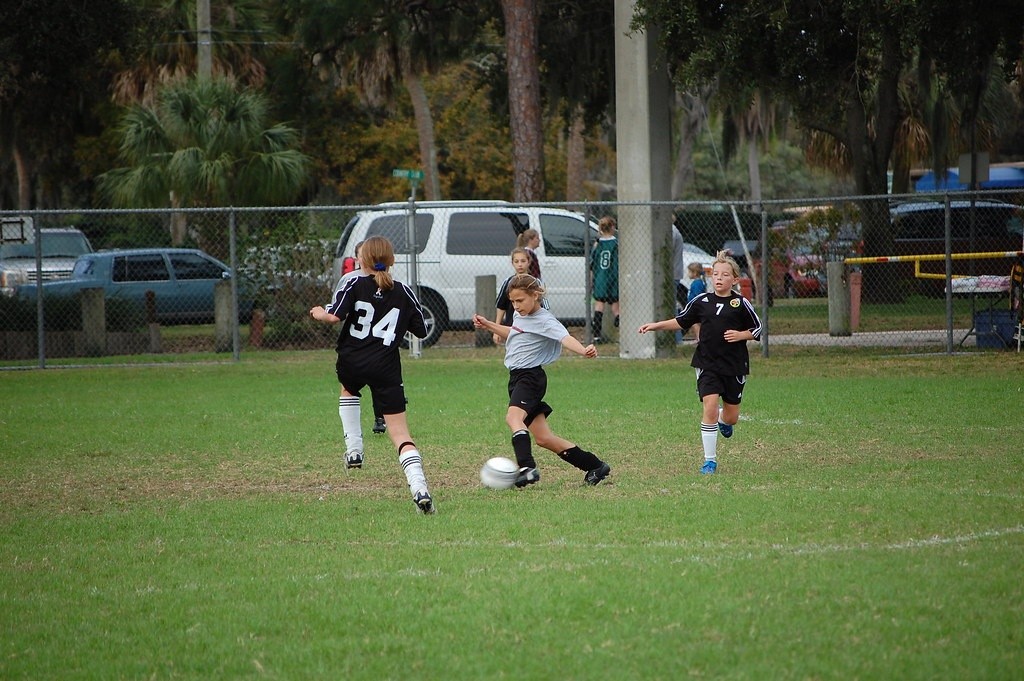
<box><xmin>479</xmin><ymin>457</ymin><xmax>519</xmax><ymax>490</ymax></box>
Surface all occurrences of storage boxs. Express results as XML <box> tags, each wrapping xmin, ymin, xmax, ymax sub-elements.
<box><xmin>974</xmin><ymin>309</ymin><xmax>1016</xmax><ymax>348</ymax></box>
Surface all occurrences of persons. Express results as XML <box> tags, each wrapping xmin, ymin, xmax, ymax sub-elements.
<box><xmin>739</xmin><ymin>256</ymin><xmax>773</xmax><ymax>308</ymax></box>
<box><xmin>492</xmin><ymin>248</ymin><xmax>531</xmax><ymax>344</ymax></box>
<box><xmin>517</xmin><ymin>229</ymin><xmax>541</xmax><ymax>280</ymax></box>
<box><xmin>473</xmin><ymin>274</ymin><xmax>610</xmax><ymax>489</ymax></box>
<box><xmin>588</xmin><ymin>215</ymin><xmax>619</xmax><ymax>344</ymax></box>
<box><xmin>672</xmin><ymin>214</ymin><xmax>684</xmax><ymax>345</ymax></box>
<box><xmin>638</xmin><ymin>251</ymin><xmax>763</xmax><ymax>476</ymax></box>
<box><xmin>310</xmin><ymin>236</ymin><xmax>438</xmax><ymax>517</ymax></box>
<box><xmin>331</xmin><ymin>241</ymin><xmax>386</xmax><ymax>434</ymax></box>
<box><xmin>687</xmin><ymin>263</ymin><xmax>708</xmax><ymax>345</ymax></box>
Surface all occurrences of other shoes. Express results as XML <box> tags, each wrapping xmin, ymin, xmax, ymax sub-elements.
<box><xmin>592</xmin><ymin>332</ymin><xmax>600</xmax><ymax>341</ymax></box>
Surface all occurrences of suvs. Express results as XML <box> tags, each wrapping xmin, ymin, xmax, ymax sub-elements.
<box><xmin>823</xmin><ymin>195</ymin><xmax>1024</xmax><ymax>298</ymax></box>
<box><xmin>0</xmin><ymin>216</ymin><xmax>98</xmax><ymax>302</ymax></box>
<box><xmin>332</xmin><ymin>197</ymin><xmax>757</xmax><ymax>350</ymax></box>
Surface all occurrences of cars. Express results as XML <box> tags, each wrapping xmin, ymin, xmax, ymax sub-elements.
<box><xmin>744</xmin><ymin>221</ymin><xmax>862</xmax><ymax>300</ymax></box>
<box><xmin>12</xmin><ymin>245</ymin><xmax>287</xmax><ymax>336</ymax></box>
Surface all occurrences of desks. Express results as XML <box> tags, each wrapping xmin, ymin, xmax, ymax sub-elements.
<box><xmin>944</xmin><ymin>274</ymin><xmax>1010</xmax><ymax>351</ymax></box>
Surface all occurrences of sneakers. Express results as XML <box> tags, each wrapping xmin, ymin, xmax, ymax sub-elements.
<box><xmin>372</xmin><ymin>417</ymin><xmax>386</xmax><ymax>433</ymax></box>
<box><xmin>718</xmin><ymin>404</ymin><xmax>732</xmax><ymax>438</ymax></box>
<box><xmin>343</xmin><ymin>449</ymin><xmax>364</xmax><ymax>469</ymax></box>
<box><xmin>412</xmin><ymin>488</ymin><xmax>435</xmax><ymax>515</ymax></box>
<box><xmin>701</xmin><ymin>461</ymin><xmax>717</xmax><ymax>476</ymax></box>
<box><xmin>515</xmin><ymin>467</ymin><xmax>539</xmax><ymax>488</ymax></box>
<box><xmin>584</xmin><ymin>462</ymin><xmax>610</xmax><ymax>486</ymax></box>
